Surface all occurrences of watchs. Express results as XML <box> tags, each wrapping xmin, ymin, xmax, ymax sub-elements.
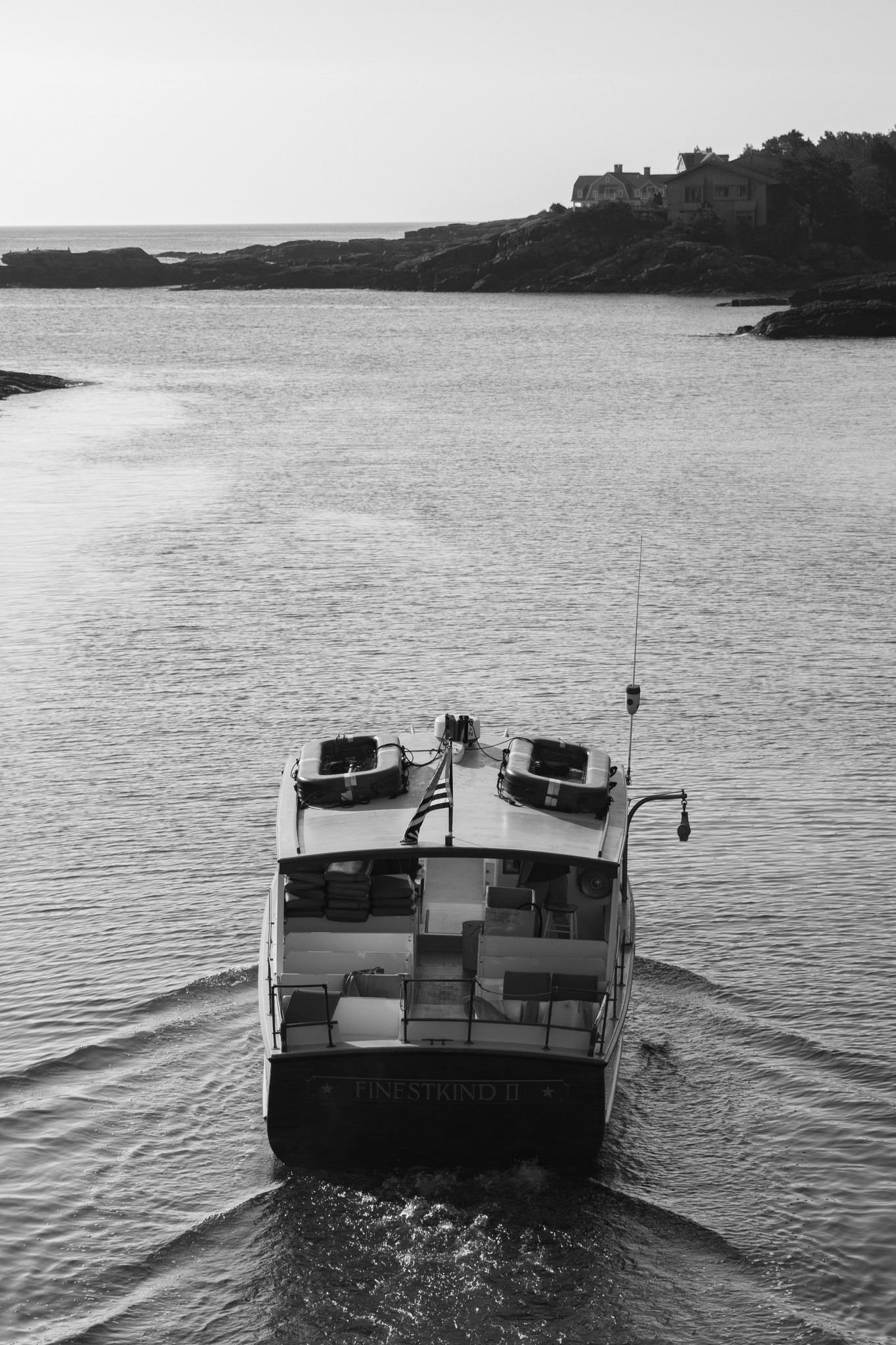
<box><xmin>517</xmin><ymin>880</ymin><xmax>525</xmax><ymax>885</ymax></box>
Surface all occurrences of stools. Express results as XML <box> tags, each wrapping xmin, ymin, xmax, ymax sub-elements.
<box><xmin>543</xmin><ymin>903</ymin><xmax>578</xmax><ymax>940</ymax></box>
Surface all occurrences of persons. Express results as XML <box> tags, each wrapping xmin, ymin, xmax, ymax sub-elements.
<box><xmin>515</xmin><ymin>860</ymin><xmax>572</xmax><ymax>940</ymax></box>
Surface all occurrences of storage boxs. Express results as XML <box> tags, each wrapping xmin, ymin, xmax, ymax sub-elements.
<box><xmin>485</xmin><ymin>886</ymin><xmax>536</xmax><ymax>938</ymax></box>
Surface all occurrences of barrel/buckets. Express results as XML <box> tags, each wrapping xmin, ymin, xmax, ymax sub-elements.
<box><xmin>461</xmin><ymin>920</ymin><xmax>484</xmax><ymax>971</ymax></box>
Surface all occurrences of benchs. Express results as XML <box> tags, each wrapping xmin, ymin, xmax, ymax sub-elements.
<box><xmin>475</xmin><ymin>933</ymin><xmax>609</xmax><ymax>1050</ymax></box>
<box><xmin>280</xmin><ymin>906</ymin><xmax>419</xmax><ymax>1037</ymax></box>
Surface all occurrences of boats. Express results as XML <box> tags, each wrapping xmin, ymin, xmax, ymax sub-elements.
<box><xmin>254</xmin><ymin>710</ymin><xmax>694</xmax><ymax>1179</ymax></box>
